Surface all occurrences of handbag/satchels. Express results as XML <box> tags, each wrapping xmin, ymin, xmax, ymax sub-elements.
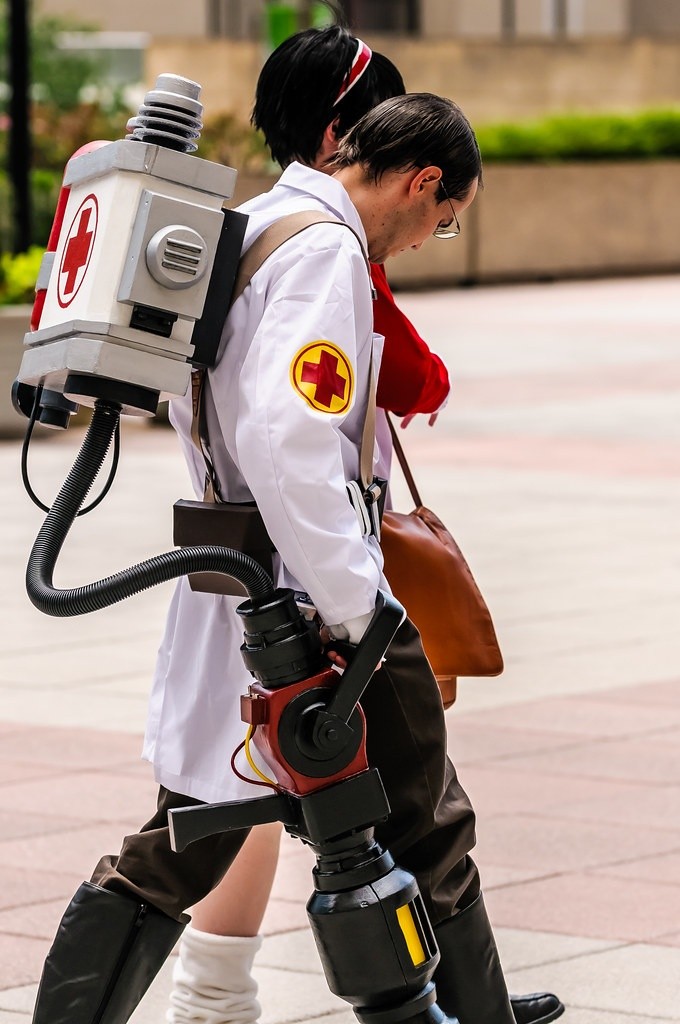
<box><xmin>377</xmin><ymin>499</ymin><xmax>505</xmax><ymax>710</ymax></box>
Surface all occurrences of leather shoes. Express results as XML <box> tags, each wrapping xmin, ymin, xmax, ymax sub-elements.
<box><xmin>508</xmin><ymin>992</ymin><xmax>565</xmax><ymax>1024</ymax></box>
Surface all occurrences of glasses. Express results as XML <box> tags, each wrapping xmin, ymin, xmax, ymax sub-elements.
<box><xmin>433</xmin><ymin>178</ymin><xmax>461</xmax><ymax>239</ymax></box>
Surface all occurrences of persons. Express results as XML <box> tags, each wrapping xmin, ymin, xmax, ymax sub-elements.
<box><xmin>166</xmin><ymin>23</ymin><xmax>565</xmax><ymax>1024</ymax></box>
<box><xmin>32</xmin><ymin>91</ymin><xmax>524</xmax><ymax>1024</ymax></box>
